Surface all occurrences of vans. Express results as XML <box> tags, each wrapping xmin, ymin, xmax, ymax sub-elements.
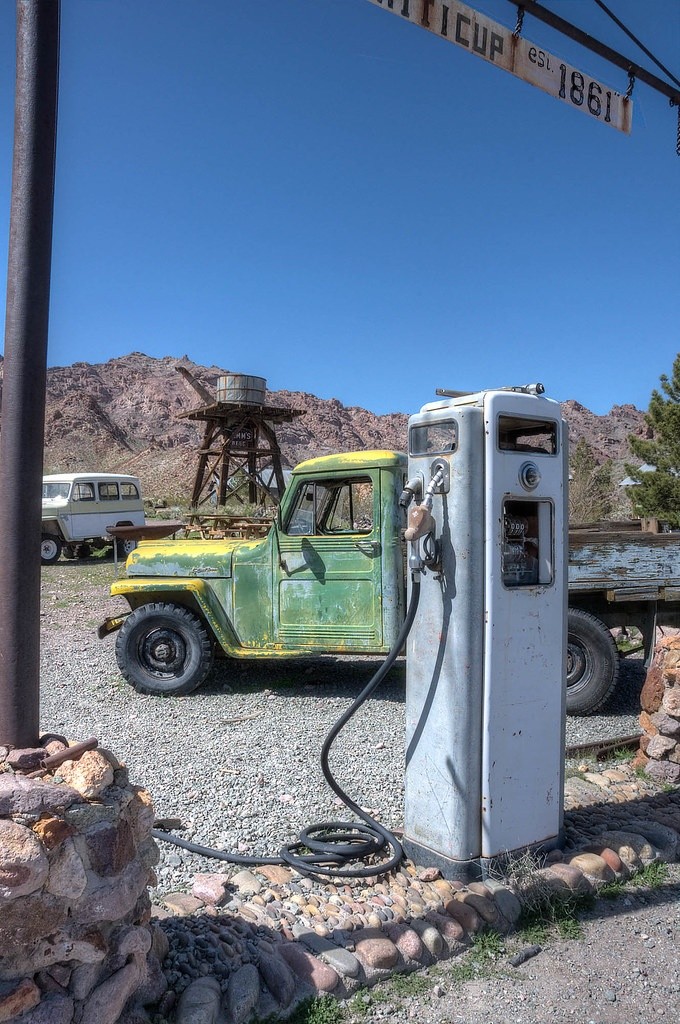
<box><xmin>42</xmin><ymin>473</ymin><xmax>147</xmax><ymax>565</ymax></box>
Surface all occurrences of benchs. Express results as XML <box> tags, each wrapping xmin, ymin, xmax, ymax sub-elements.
<box><xmin>182</xmin><ymin>524</ymin><xmax>267</xmax><ymax>540</ymax></box>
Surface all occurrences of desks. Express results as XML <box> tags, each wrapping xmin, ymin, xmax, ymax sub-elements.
<box><xmin>234</xmin><ymin>522</ymin><xmax>272</xmax><ymax>539</ymax></box>
<box><xmin>183</xmin><ymin>513</ymin><xmax>247</xmax><ymax>540</ymax></box>
<box><xmin>203</xmin><ymin>516</ymin><xmax>273</xmax><ymax>539</ymax></box>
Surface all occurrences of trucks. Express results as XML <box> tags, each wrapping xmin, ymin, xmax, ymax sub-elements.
<box><xmin>97</xmin><ymin>450</ymin><xmax>680</xmax><ymax>717</ymax></box>
